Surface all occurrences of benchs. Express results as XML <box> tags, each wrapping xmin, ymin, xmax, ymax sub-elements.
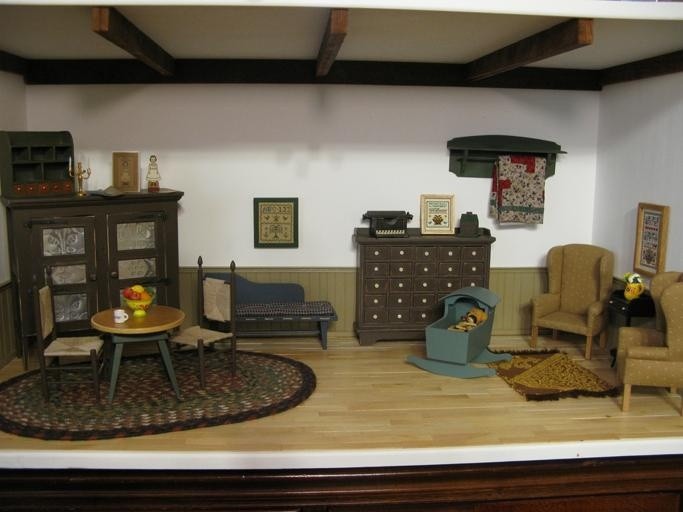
<box><xmin>203</xmin><ymin>272</ymin><xmax>338</xmax><ymax>351</ymax></box>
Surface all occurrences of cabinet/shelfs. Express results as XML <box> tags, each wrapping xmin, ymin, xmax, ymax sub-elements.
<box><xmin>0</xmin><ymin>128</ymin><xmax>76</xmax><ymax>202</ymax></box>
<box><xmin>0</xmin><ymin>181</ymin><xmax>188</xmax><ymax>359</ymax></box>
<box><xmin>349</xmin><ymin>224</ymin><xmax>497</xmax><ymax>349</ymax></box>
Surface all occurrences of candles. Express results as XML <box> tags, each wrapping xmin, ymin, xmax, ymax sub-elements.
<box><xmin>67</xmin><ymin>150</ymin><xmax>92</xmax><ymax>173</ymax></box>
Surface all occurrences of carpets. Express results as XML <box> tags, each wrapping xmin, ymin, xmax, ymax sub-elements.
<box><xmin>480</xmin><ymin>342</ymin><xmax>625</xmax><ymax>406</ymax></box>
<box><xmin>0</xmin><ymin>348</ymin><xmax>319</xmax><ymax>443</ymax></box>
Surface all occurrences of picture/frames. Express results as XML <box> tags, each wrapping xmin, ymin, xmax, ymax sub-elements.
<box><xmin>251</xmin><ymin>196</ymin><xmax>298</xmax><ymax>250</ymax></box>
<box><xmin>631</xmin><ymin>201</ymin><xmax>670</xmax><ymax>279</ymax></box>
<box><xmin>110</xmin><ymin>150</ymin><xmax>142</xmax><ymax>193</ymax></box>
<box><xmin>418</xmin><ymin>193</ymin><xmax>457</xmax><ymax>236</ymax></box>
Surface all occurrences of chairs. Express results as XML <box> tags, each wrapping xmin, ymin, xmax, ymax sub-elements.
<box><xmin>527</xmin><ymin>243</ymin><xmax>617</xmax><ymax>362</ymax></box>
<box><xmin>28</xmin><ymin>264</ymin><xmax>112</xmax><ymax>406</ymax></box>
<box><xmin>614</xmin><ymin>269</ymin><xmax>683</xmax><ymax>414</ymax></box>
<box><xmin>156</xmin><ymin>253</ymin><xmax>239</xmax><ymax>390</ymax></box>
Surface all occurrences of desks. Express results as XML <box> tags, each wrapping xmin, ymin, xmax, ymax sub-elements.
<box><xmin>86</xmin><ymin>303</ymin><xmax>189</xmax><ymax>406</ymax></box>
<box><xmin>604</xmin><ymin>288</ymin><xmax>656</xmax><ymax>369</ymax></box>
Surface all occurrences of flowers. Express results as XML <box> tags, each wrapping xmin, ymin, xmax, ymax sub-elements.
<box><xmin>619</xmin><ymin>271</ymin><xmax>645</xmax><ymax>286</ymax></box>
